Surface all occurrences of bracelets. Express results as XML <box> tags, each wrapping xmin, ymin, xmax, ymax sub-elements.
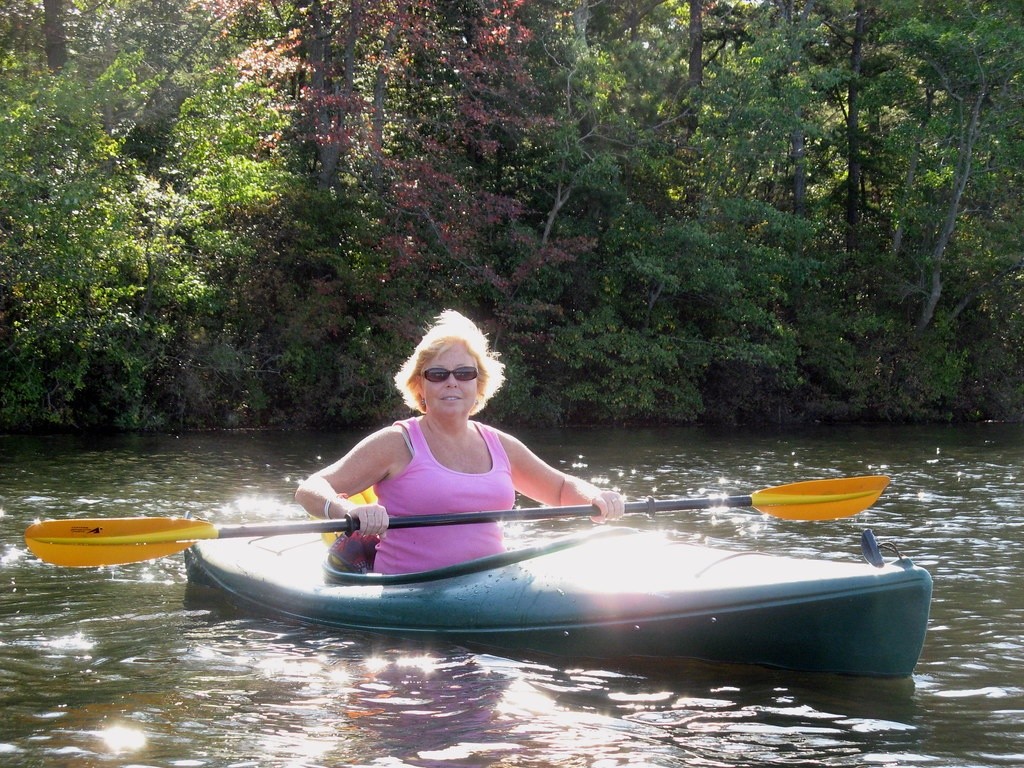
<box><xmin>324</xmin><ymin>494</ymin><xmax>344</xmax><ymax>520</ymax></box>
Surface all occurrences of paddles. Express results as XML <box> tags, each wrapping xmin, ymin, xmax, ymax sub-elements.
<box><xmin>25</xmin><ymin>475</ymin><xmax>892</xmax><ymax>567</ymax></box>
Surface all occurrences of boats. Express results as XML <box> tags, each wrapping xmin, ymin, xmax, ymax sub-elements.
<box><xmin>184</xmin><ymin>509</ymin><xmax>933</xmax><ymax>678</ymax></box>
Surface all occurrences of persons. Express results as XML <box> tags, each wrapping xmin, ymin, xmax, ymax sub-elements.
<box><xmin>296</xmin><ymin>310</ymin><xmax>624</xmax><ymax>575</ymax></box>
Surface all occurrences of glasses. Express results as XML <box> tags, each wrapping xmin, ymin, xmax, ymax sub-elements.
<box><xmin>418</xmin><ymin>366</ymin><xmax>479</xmax><ymax>383</ymax></box>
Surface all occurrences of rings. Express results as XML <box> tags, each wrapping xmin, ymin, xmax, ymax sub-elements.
<box><xmin>612</xmin><ymin>498</ymin><xmax>617</xmax><ymax>502</ymax></box>
<box><xmin>368</xmin><ymin>514</ymin><xmax>374</xmax><ymax>516</ymax></box>
